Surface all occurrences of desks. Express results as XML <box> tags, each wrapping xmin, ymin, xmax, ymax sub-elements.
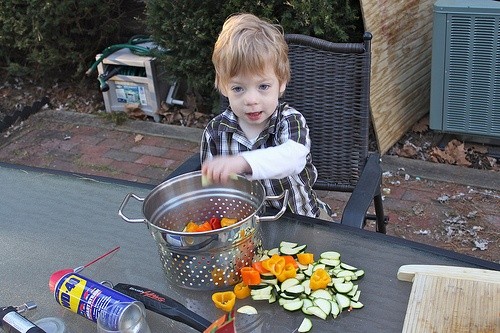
<box><xmin>1</xmin><ymin>162</ymin><xmax>499</xmax><ymax>332</ymax></box>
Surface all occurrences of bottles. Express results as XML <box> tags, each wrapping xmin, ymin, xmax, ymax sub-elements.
<box><xmin>0</xmin><ymin>305</ymin><xmax>46</xmax><ymax>333</ymax></box>
<box><xmin>47</xmin><ymin>268</ymin><xmax>145</xmax><ymax>332</ymax></box>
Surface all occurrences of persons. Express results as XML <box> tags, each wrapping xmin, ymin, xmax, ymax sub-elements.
<box><xmin>199</xmin><ymin>12</ymin><xmax>337</xmax><ymax>224</ymax></box>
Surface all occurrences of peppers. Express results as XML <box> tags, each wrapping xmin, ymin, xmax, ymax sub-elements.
<box><xmin>182</xmin><ymin>217</ymin><xmax>237</xmax><ymax>234</ymax></box>
<box><xmin>211</xmin><ymin>252</ymin><xmax>331</xmax><ymax>312</ymax></box>
<box><xmin>212</xmin><ymin>234</ymin><xmax>255</xmax><ymax>284</ymax></box>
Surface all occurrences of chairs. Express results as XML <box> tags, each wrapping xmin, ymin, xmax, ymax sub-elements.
<box><xmin>162</xmin><ymin>33</ymin><xmax>388</xmax><ymax>235</ymax></box>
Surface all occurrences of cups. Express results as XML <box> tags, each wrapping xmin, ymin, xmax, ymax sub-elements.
<box><xmin>96</xmin><ymin>302</ymin><xmax>151</xmax><ymax>333</ymax></box>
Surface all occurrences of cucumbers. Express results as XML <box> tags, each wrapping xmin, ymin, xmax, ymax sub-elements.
<box><xmin>237</xmin><ymin>241</ymin><xmax>364</xmax><ymax>332</ymax></box>
